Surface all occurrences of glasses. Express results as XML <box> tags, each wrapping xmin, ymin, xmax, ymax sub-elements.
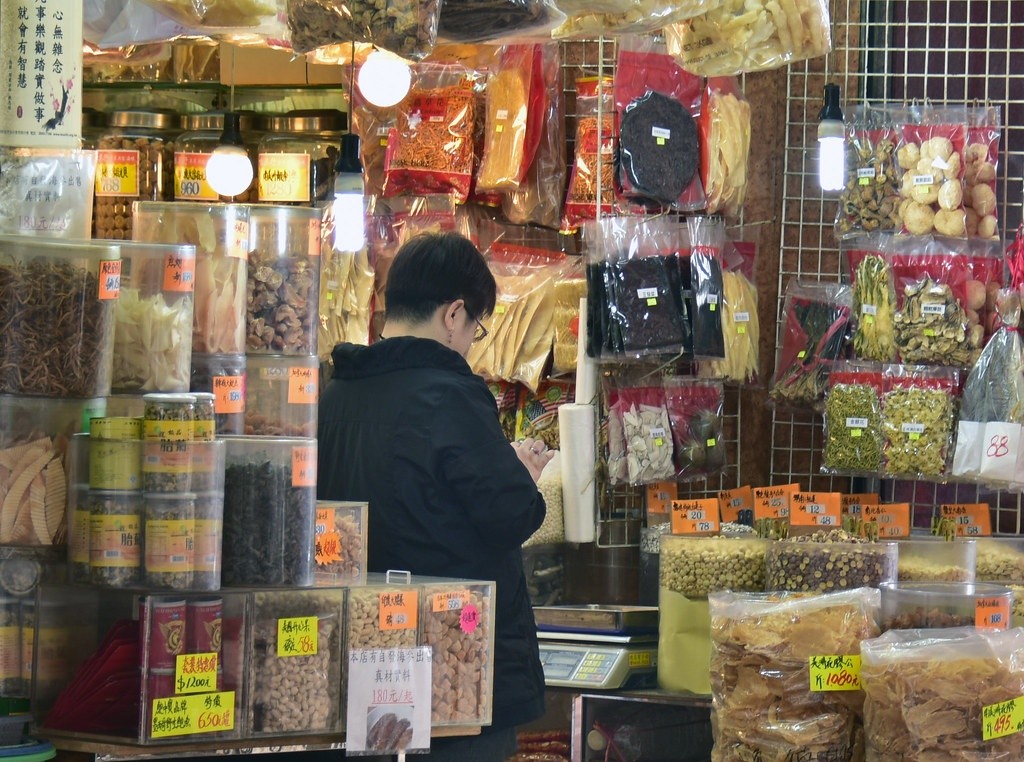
<box><xmin>439</xmin><ymin>297</ymin><xmax>489</xmax><ymax>346</ymax></box>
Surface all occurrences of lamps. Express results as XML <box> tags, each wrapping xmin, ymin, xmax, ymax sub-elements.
<box><xmin>333</xmin><ymin>39</ymin><xmax>368</xmax><ymax>253</ymax></box>
<box><xmin>206</xmin><ymin>43</ymin><xmax>254</xmax><ymax>199</ymax></box>
<box><xmin>818</xmin><ymin>0</ymin><xmax>846</xmax><ymax>190</ymax></box>
<box><xmin>357</xmin><ymin>46</ymin><xmax>412</xmax><ymax>108</ymax></box>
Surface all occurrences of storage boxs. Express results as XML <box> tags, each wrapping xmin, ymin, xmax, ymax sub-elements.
<box><xmin>307</xmin><ymin>52</ymin><xmax>342</xmax><ymax>87</ymax></box>
<box><xmin>218</xmin><ymin>40</ymin><xmax>307</xmax><ymax>86</ymax></box>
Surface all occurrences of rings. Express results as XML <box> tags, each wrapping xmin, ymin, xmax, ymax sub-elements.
<box><xmin>531</xmin><ymin>448</ymin><xmax>540</xmax><ymax>454</ymax></box>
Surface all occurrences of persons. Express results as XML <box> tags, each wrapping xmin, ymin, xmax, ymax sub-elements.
<box><xmin>315</xmin><ymin>234</ymin><xmax>556</xmax><ymax>762</ymax></box>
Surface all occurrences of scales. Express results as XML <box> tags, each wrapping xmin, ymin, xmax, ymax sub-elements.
<box><xmin>532</xmin><ymin>603</ymin><xmax>660</xmax><ymax>690</ymax></box>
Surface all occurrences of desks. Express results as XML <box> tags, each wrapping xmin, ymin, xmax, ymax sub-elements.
<box><xmin>572</xmin><ymin>688</ymin><xmax>713</xmax><ymax>762</ymax></box>
<box><xmin>31</xmin><ymin>725</ymin><xmax>407</xmax><ymax>762</ymax></box>
